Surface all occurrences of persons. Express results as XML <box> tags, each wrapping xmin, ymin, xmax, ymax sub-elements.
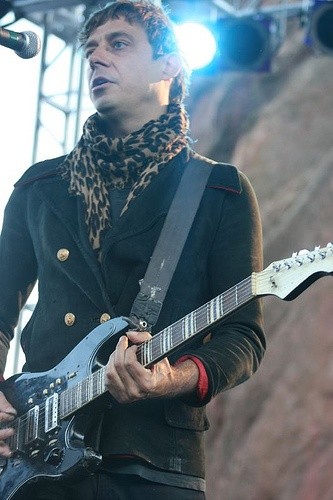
<box><xmin>0</xmin><ymin>0</ymin><xmax>268</xmax><ymax>500</ymax></box>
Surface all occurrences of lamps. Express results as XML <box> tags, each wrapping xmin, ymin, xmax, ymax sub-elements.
<box><xmin>305</xmin><ymin>0</ymin><xmax>333</xmax><ymax>56</ymax></box>
<box><xmin>218</xmin><ymin>14</ymin><xmax>284</xmax><ymax>74</ymax></box>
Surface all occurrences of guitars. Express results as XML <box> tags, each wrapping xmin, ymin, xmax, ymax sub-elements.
<box><xmin>0</xmin><ymin>242</ymin><xmax>333</xmax><ymax>500</ymax></box>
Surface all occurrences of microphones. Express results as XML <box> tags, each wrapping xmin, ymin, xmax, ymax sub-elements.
<box><xmin>0</xmin><ymin>28</ymin><xmax>40</xmax><ymax>59</ymax></box>
